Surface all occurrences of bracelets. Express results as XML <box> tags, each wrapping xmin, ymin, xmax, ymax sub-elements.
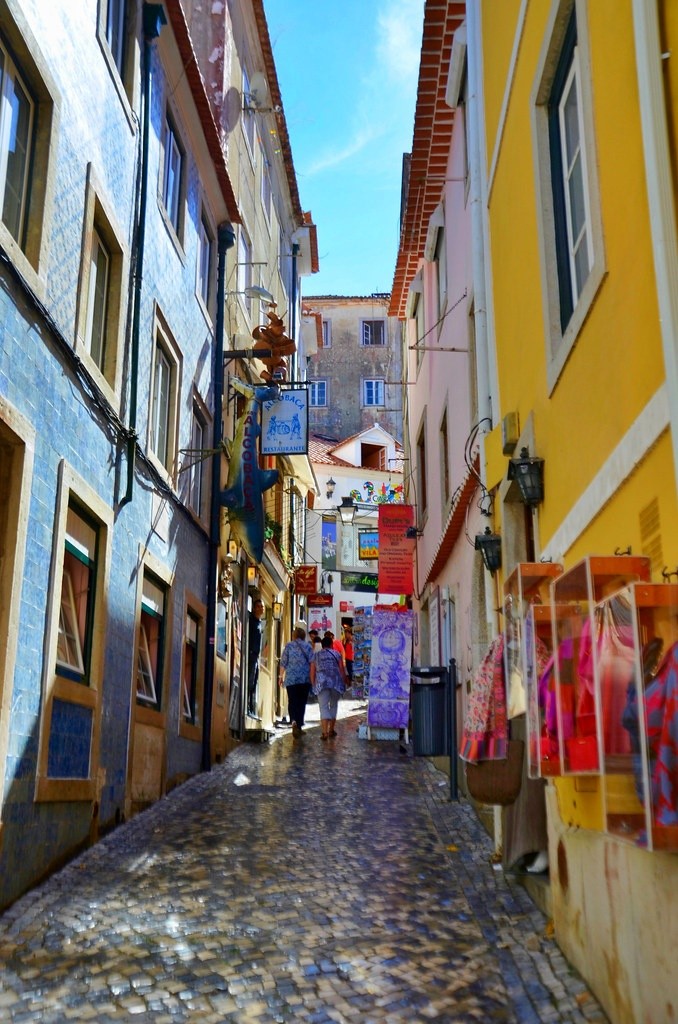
<box><xmin>343</xmin><ymin>667</ymin><xmax>348</xmax><ymax>671</ymax></box>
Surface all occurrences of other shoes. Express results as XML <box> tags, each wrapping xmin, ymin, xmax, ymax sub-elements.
<box><xmin>329</xmin><ymin>730</ymin><xmax>337</xmax><ymax>737</ymax></box>
<box><xmin>298</xmin><ymin>729</ymin><xmax>306</xmax><ymax>735</ymax></box>
<box><xmin>292</xmin><ymin>722</ymin><xmax>298</xmax><ymax>738</ymax></box>
<box><xmin>320</xmin><ymin>733</ymin><xmax>328</xmax><ymax>740</ymax></box>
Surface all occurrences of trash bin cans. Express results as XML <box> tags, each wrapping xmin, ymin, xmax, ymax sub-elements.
<box><xmin>408</xmin><ymin>664</ymin><xmax>448</xmax><ymax>756</ymax></box>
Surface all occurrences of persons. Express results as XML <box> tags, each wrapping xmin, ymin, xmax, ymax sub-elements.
<box><xmin>305</xmin><ymin>630</ymin><xmax>318</xmax><ymax>702</ymax></box>
<box><xmin>323</xmin><ymin>630</ymin><xmax>351</xmax><ymax>686</ymax></box>
<box><xmin>278</xmin><ymin>626</ymin><xmax>316</xmax><ymax>738</ymax></box>
<box><xmin>314</xmin><ymin>636</ymin><xmax>349</xmax><ymax>740</ymax></box>
<box><xmin>248</xmin><ymin>598</ymin><xmax>265</xmax><ymax>716</ymax></box>
<box><xmin>342</xmin><ymin>628</ymin><xmax>354</xmax><ymax>682</ymax></box>
